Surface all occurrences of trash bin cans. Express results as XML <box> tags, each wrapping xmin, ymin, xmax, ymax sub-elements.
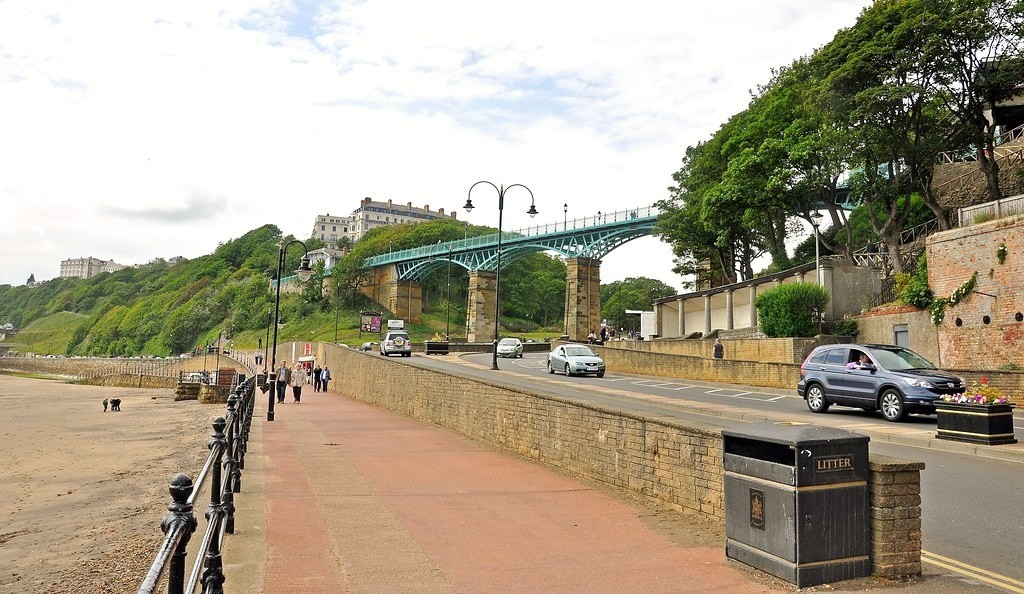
<box><xmin>725</xmin><ymin>421</ymin><xmax>873</xmax><ymax>587</ymax></box>
<box><xmin>255</xmin><ymin>355</ymin><xmax>263</xmax><ymax>364</ymax></box>
<box><xmin>257</xmin><ymin>374</ymin><xmax>264</xmax><ymax>386</ymax></box>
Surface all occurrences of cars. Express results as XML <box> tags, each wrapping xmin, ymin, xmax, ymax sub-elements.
<box><xmin>546</xmin><ymin>344</ymin><xmax>606</xmax><ymax>378</ymax></box>
<box><xmin>359</xmin><ymin>341</ymin><xmax>378</xmax><ymax>352</ymax></box>
<box><xmin>497</xmin><ymin>337</ymin><xmax>524</xmax><ymax>359</ymax></box>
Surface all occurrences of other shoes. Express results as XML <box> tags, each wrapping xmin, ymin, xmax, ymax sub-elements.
<box><xmin>298</xmin><ymin>400</ymin><xmax>300</xmax><ymax>404</ymax></box>
<box><xmin>278</xmin><ymin>400</ymin><xmax>281</xmax><ymax>403</ymax></box>
<box><xmin>293</xmin><ymin>400</ymin><xmax>297</xmax><ymax>403</ymax></box>
<box><xmin>281</xmin><ymin>401</ymin><xmax>284</xmax><ymax>404</ymax></box>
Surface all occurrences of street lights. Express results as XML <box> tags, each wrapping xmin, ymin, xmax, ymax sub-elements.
<box><xmin>428</xmin><ymin>243</ymin><xmax>473</xmax><ymax>356</ymax></box>
<box><xmin>214</xmin><ymin>329</ymin><xmax>231</xmax><ymax>385</ymax></box>
<box><xmin>261</xmin><ymin>308</ymin><xmax>288</xmax><ymax>395</ymax></box>
<box><xmin>266</xmin><ymin>239</ymin><xmax>316</xmax><ymax>423</ymax></box>
<box><xmin>809</xmin><ymin>211</ymin><xmax>824</xmax><ymax>335</ymax></box>
<box><xmin>563</xmin><ymin>203</ymin><xmax>568</xmax><ymax>231</ymax></box>
<box><xmin>463</xmin><ymin>179</ymin><xmax>539</xmax><ymax>372</ymax></box>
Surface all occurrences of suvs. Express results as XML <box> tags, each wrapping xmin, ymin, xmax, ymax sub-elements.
<box><xmin>377</xmin><ymin>328</ymin><xmax>412</xmax><ymax>358</ymax></box>
<box><xmin>796</xmin><ymin>343</ymin><xmax>968</xmax><ymax>424</ymax></box>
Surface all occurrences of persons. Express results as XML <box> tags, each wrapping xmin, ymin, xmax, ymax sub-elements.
<box><xmin>589</xmin><ymin>331</ymin><xmax>596</xmax><ymax>338</ymax></box>
<box><xmin>314</xmin><ymin>366</ymin><xmax>322</xmax><ymax>392</ymax></box>
<box><xmin>712</xmin><ymin>338</ymin><xmax>724</xmax><ymax>358</ymax></box>
<box><xmin>258</xmin><ymin>338</ymin><xmax>262</xmax><ymax>349</ymax></box>
<box><xmin>290</xmin><ymin>363</ymin><xmax>306</xmax><ymax>404</ymax></box>
<box><xmin>863</xmin><ymin>237</ymin><xmax>875</xmax><ymax>258</ymax></box>
<box><xmin>255</xmin><ymin>351</ymin><xmax>263</xmax><ymax>365</ymax></box>
<box><xmin>600</xmin><ymin>327</ymin><xmax>615</xmax><ymax>341</ymax></box>
<box><xmin>880</xmin><ymin>242</ymin><xmax>889</xmax><ymax>263</ymax></box>
<box><xmin>110</xmin><ymin>398</ymin><xmax>121</xmax><ymax>411</ymax></box>
<box><xmin>103</xmin><ymin>397</ymin><xmax>108</xmax><ymax>412</ymax></box>
<box><xmin>192</xmin><ymin>347</ymin><xmax>199</xmax><ymax>357</ymax></box>
<box><xmin>275</xmin><ymin>360</ymin><xmax>291</xmax><ymax>404</ymax></box>
<box><xmin>320</xmin><ymin>366</ymin><xmax>331</xmax><ymax>393</ymax></box>
<box><xmin>848</xmin><ymin>353</ymin><xmax>869</xmax><ymax>369</ymax></box>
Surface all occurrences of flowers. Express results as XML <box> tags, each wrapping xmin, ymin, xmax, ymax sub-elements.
<box><xmin>940</xmin><ymin>376</ymin><xmax>1010</xmax><ymax>406</ymax></box>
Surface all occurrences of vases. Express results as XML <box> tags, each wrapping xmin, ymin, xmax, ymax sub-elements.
<box><xmin>257</xmin><ymin>375</ymin><xmax>264</xmax><ymax>386</ymax></box>
<box><xmin>933</xmin><ymin>400</ymin><xmax>1018</xmax><ymax>446</ymax></box>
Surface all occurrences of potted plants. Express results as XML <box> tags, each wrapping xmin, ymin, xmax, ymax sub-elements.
<box><xmin>424</xmin><ymin>332</ymin><xmax>449</xmax><ymax>355</ymax></box>
